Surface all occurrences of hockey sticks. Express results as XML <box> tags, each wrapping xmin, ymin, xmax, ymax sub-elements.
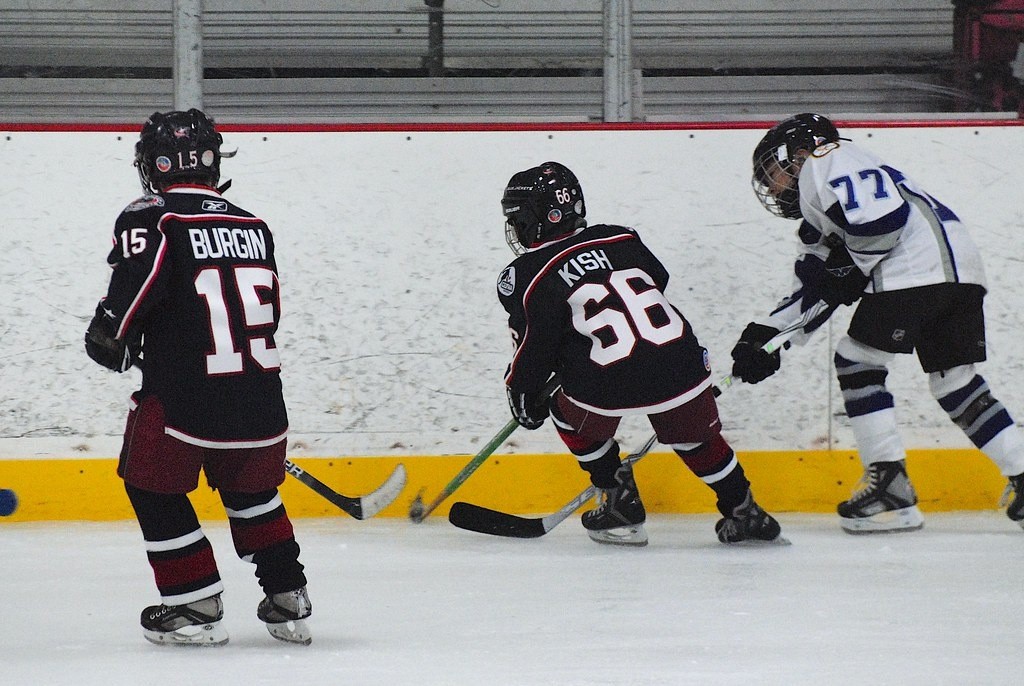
<box><xmin>448</xmin><ymin>294</ymin><xmax>829</xmax><ymax>536</ymax></box>
<box><xmin>411</xmin><ymin>374</ymin><xmax>559</xmax><ymax>523</ymax></box>
<box><xmin>136</xmin><ymin>353</ymin><xmax>409</xmax><ymax>525</ymax></box>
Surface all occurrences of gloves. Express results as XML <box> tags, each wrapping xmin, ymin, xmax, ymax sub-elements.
<box><xmin>731</xmin><ymin>321</ymin><xmax>780</xmax><ymax>385</ymax></box>
<box><xmin>794</xmin><ymin>254</ymin><xmax>848</xmax><ymax>309</ymax></box>
<box><xmin>84</xmin><ymin>331</ymin><xmax>141</xmax><ymax>373</ymax></box>
<box><xmin>506</xmin><ymin>387</ymin><xmax>550</xmax><ymax>430</ymax></box>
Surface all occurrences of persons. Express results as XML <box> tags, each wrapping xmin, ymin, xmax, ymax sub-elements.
<box><xmin>84</xmin><ymin>108</ymin><xmax>312</xmax><ymax>647</ymax></box>
<box><xmin>731</xmin><ymin>113</ymin><xmax>1024</xmax><ymax>536</ymax></box>
<box><xmin>496</xmin><ymin>161</ymin><xmax>791</xmax><ymax>547</ymax></box>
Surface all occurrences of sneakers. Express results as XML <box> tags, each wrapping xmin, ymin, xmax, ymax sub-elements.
<box><xmin>998</xmin><ymin>472</ymin><xmax>1024</xmax><ymax>527</ymax></box>
<box><xmin>837</xmin><ymin>458</ymin><xmax>925</xmax><ymax>534</ymax></box>
<box><xmin>715</xmin><ymin>491</ymin><xmax>782</xmax><ymax>548</ymax></box>
<box><xmin>140</xmin><ymin>592</ymin><xmax>230</xmax><ymax>646</ymax></box>
<box><xmin>255</xmin><ymin>587</ymin><xmax>314</xmax><ymax>645</ymax></box>
<box><xmin>582</xmin><ymin>462</ymin><xmax>650</xmax><ymax>546</ymax></box>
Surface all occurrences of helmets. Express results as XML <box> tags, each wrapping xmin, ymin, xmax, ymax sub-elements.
<box><xmin>753</xmin><ymin>113</ymin><xmax>840</xmax><ymax>219</ymax></box>
<box><xmin>502</xmin><ymin>162</ymin><xmax>587</xmax><ymax>247</ymax></box>
<box><xmin>134</xmin><ymin>109</ymin><xmax>223</xmax><ymax>194</ymax></box>
